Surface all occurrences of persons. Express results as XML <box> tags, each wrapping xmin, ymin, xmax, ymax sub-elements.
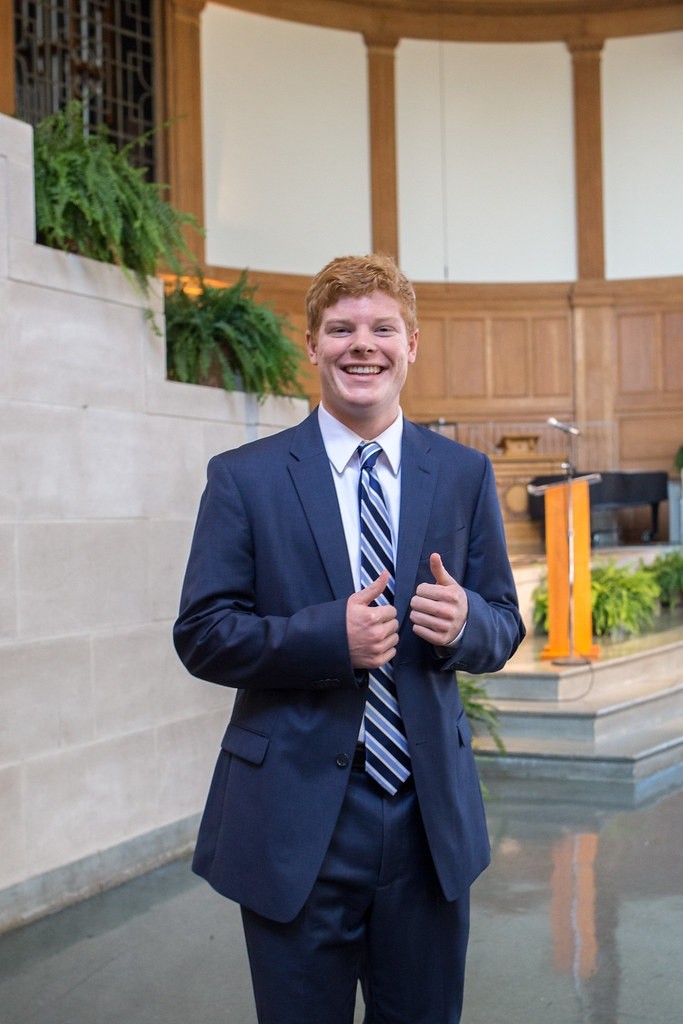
<box><xmin>173</xmin><ymin>255</ymin><xmax>528</xmax><ymax>1024</ymax></box>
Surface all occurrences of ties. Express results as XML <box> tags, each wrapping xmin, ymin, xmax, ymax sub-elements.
<box><xmin>357</xmin><ymin>441</ymin><xmax>412</xmax><ymax>796</ymax></box>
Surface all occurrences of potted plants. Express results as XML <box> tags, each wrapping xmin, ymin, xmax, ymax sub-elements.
<box><xmin>161</xmin><ymin>264</ymin><xmax>314</xmax><ymax>406</ymax></box>
<box><xmin>529</xmin><ymin>546</ymin><xmax>683</xmax><ymax>641</ymax></box>
<box><xmin>35</xmin><ymin>98</ymin><xmax>211</xmax><ymax>307</ymax></box>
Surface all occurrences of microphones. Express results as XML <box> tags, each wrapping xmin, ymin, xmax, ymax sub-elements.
<box><xmin>548</xmin><ymin>417</ymin><xmax>579</xmax><ymax>436</ymax></box>
